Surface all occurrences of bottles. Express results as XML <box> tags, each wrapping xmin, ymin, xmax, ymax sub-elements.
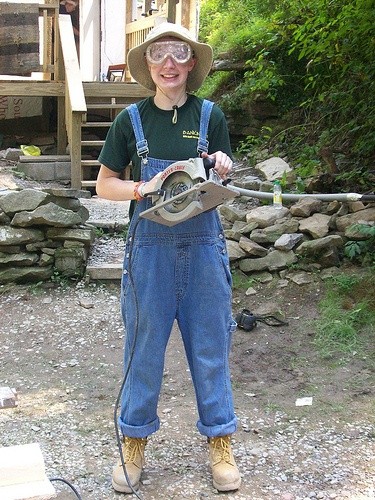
<box><xmin>273</xmin><ymin>180</ymin><xmax>282</xmax><ymax>209</ymax></box>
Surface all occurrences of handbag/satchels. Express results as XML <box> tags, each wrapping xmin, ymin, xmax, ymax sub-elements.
<box><xmin>235</xmin><ymin>313</ymin><xmax>256</xmax><ymax>331</ymax></box>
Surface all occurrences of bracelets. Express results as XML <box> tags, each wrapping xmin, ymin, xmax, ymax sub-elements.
<box><xmin>134</xmin><ymin>181</ymin><xmax>146</xmax><ymax>201</ymax></box>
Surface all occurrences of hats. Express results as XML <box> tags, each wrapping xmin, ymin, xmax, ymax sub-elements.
<box><xmin>127</xmin><ymin>23</ymin><xmax>213</xmax><ymax>93</ymax></box>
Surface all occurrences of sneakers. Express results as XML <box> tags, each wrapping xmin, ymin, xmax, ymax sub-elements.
<box><xmin>207</xmin><ymin>434</ymin><xmax>241</xmax><ymax>491</ymax></box>
<box><xmin>112</xmin><ymin>434</ymin><xmax>148</xmax><ymax>493</ymax></box>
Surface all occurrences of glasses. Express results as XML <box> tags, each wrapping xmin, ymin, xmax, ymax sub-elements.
<box><xmin>146</xmin><ymin>41</ymin><xmax>193</xmax><ymax>64</ymax></box>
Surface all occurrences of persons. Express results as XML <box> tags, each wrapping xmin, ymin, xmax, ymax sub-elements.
<box><xmin>96</xmin><ymin>22</ymin><xmax>241</xmax><ymax>494</ymax></box>
